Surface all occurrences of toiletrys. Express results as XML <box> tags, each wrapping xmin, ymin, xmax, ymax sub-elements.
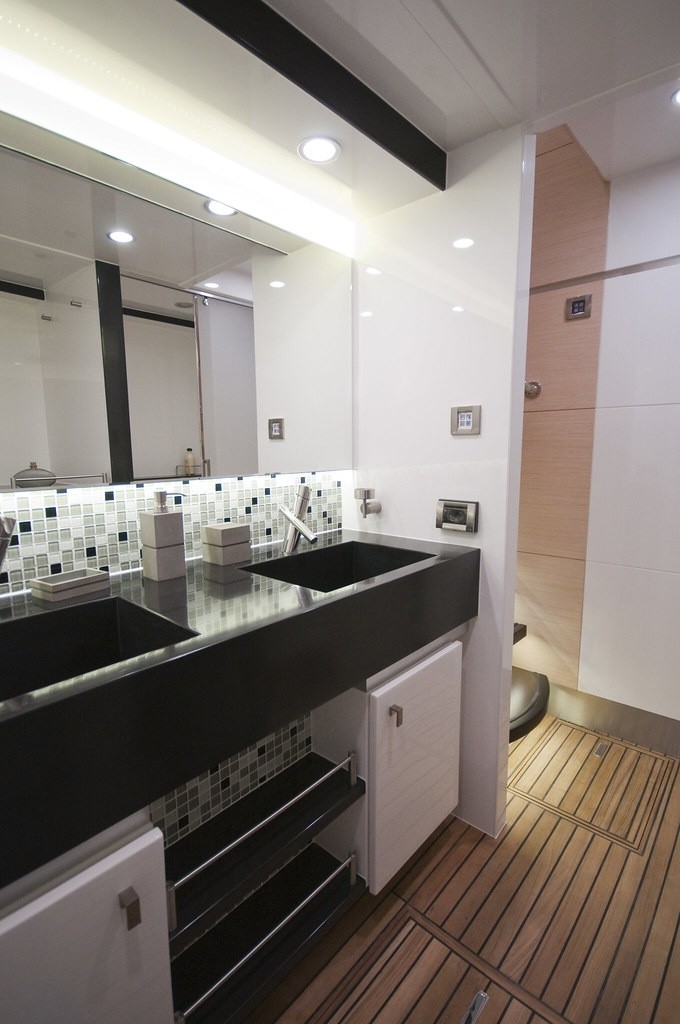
<box><xmin>184</xmin><ymin>447</ymin><xmax>196</xmax><ymax>477</ymax></box>
<box><xmin>139</xmin><ymin>490</ymin><xmax>187</xmax><ymax>582</ymax></box>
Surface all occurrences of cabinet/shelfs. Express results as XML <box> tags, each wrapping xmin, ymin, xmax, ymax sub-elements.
<box><xmin>0</xmin><ymin>806</ymin><xmax>174</xmax><ymax>1024</ymax></box>
<box><xmin>309</xmin><ymin>622</ymin><xmax>470</xmax><ymax>895</ymax></box>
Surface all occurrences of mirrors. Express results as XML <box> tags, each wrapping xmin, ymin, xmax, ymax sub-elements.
<box><xmin>0</xmin><ymin>110</ymin><xmax>356</xmax><ymax>495</ymax></box>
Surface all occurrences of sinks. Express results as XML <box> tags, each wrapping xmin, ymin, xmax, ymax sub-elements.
<box><xmin>0</xmin><ymin>595</ymin><xmax>202</xmax><ymax>704</ymax></box>
<box><xmin>237</xmin><ymin>540</ymin><xmax>438</xmax><ymax>594</ymax></box>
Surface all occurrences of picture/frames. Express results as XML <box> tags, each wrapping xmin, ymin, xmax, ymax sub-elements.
<box><xmin>566</xmin><ymin>294</ymin><xmax>592</xmax><ymax>320</ymax></box>
<box><xmin>268</xmin><ymin>418</ymin><xmax>284</xmax><ymax>439</ymax></box>
<box><xmin>451</xmin><ymin>406</ymin><xmax>481</xmax><ymax>435</ymax></box>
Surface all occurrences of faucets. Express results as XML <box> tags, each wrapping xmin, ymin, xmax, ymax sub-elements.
<box><xmin>279</xmin><ymin>484</ymin><xmax>318</xmax><ymax>555</ymax></box>
<box><xmin>0</xmin><ymin>516</ymin><xmax>16</xmax><ymax>579</ymax></box>
